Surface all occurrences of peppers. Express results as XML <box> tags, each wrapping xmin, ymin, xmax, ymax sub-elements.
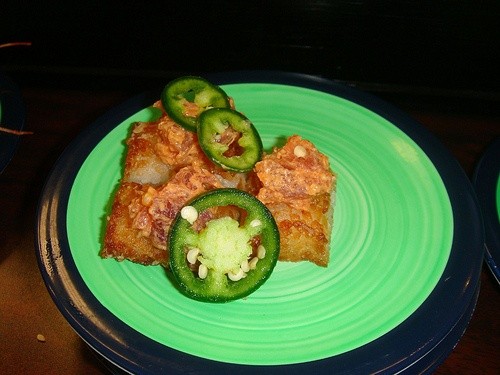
<box><xmin>162</xmin><ymin>75</ymin><xmax>231</xmax><ymax>132</ymax></box>
<box><xmin>168</xmin><ymin>187</ymin><xmax>281</xmax><ymax>303</ymax></box>
<box><xmin>196</xmin><ymin>107</ymin><xmax>263</xmax><ymax>173</ymax></box>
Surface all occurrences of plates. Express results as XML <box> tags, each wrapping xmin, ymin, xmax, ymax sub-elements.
<box><xmin>471</xmin><ymin>138</ymin><xmax>499</xmax><ymax>288</ymax></box>
<box><xmin>0</xmin><ymin>37</ymin><xmax>30</xmax><ymax>174</ymax></box>
<box><xmin>32</xmin><ymin>70</ymin><xmax>486</xmax><ymax>375</ymax></box>
